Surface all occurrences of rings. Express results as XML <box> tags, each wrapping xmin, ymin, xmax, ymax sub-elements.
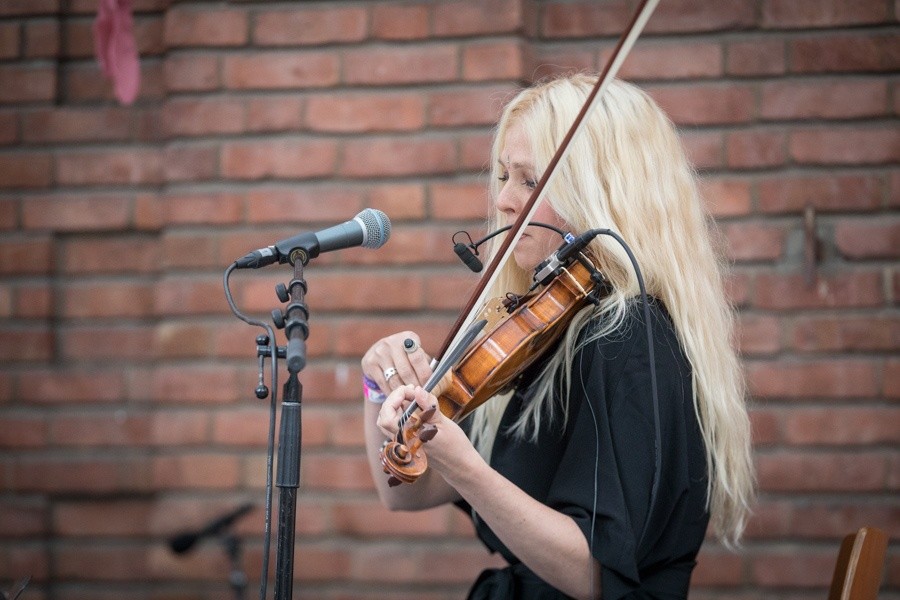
<box><xmin>403</xmin><ymin>337</ymin><xmax>417</xmax><ymax>353</ymax></box>
<box><xmin>383</xmin><ymin>367</ymin><xmax>399</xmax><ymax>382</ymax></box>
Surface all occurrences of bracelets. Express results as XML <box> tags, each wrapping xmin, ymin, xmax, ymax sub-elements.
<box><xmin>362</xmin><ymin>376</ymin><xmax>387</xmax><ymax>403</ymax></box>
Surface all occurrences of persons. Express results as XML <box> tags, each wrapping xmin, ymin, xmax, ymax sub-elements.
<box><xmin>361</xmin><ymin>73</ymin><xmax>759</xmax><ymax>600</ymax></box>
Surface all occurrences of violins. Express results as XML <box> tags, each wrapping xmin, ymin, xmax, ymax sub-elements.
<box><xmin>379</xmin><ymin>250</ymin><xmax>610</xmax><ymax>488</ymax></box>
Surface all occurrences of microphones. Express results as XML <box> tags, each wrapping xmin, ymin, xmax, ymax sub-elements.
<box><xmin>451</xmin><ymin>230</ymin><xmax>483</xmax><ymax>272</ymax></box>
<box><xmin>234</xmin><ymin>209</ymin><xmax>391</xmax><ymax>270</ymax></box>
